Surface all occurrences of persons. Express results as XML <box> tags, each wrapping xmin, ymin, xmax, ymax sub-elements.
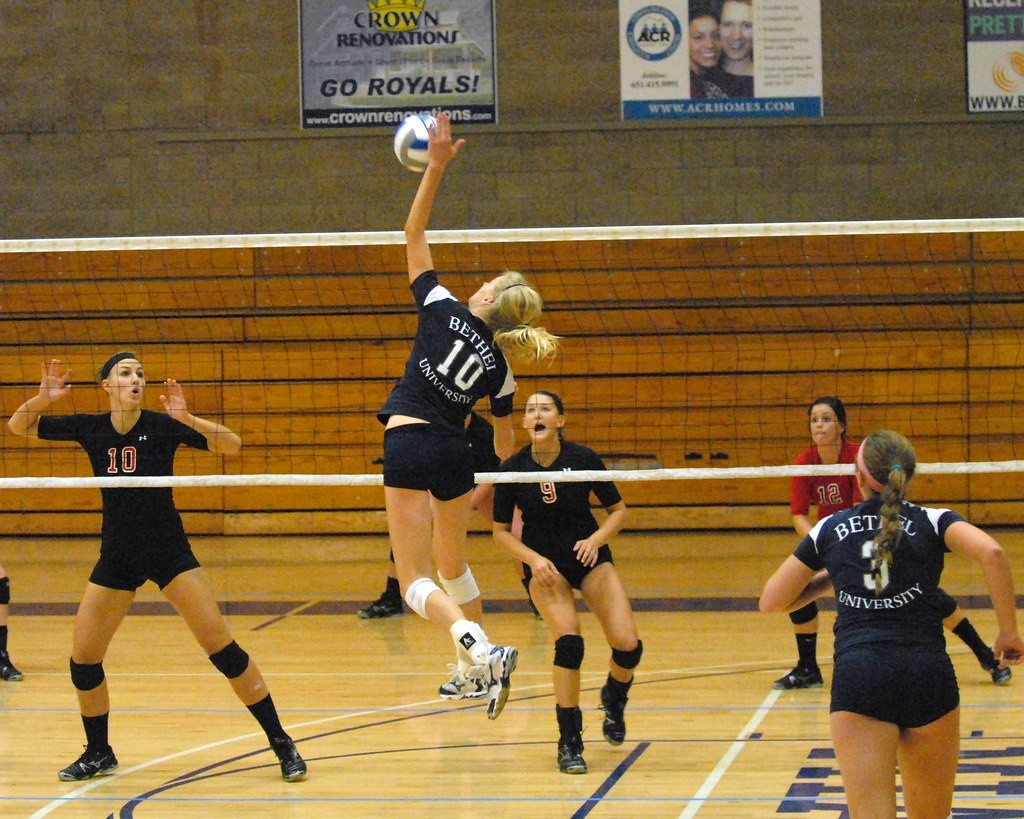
<box><xmin>492</xmin><ymin>391</ymin><xmax>643</xmax><ymax>772</ymax></box>
<box><xmin>686</xmin><ymin>1</ymin><xmax>756</xmax><ymax>99</ymax></box>
<box><xmin>373</xmin><ymin>111</ymin><xmax>560</xmax><ymax>722</ymax></box>
<box><xmin>0</xmin><ymin>565</ymin><xmax>23</xmax><ymax>684</ymax></box>
<box><xmin>8</xmin><ymin>351</ymin><xmax>310</xmax><ymax>786</ymax></box>
<box><xmin>772</xmin><ymin>400</ymin><xmax>1011</xmax><ymax>693</ymax></box>
<box><xmin>358</xmin><ymin>407</ymin><xmax>544</xmax><ymax>621</ymax></box>
<box><xmin>757</xmin><ymin>428</ymin><xmax>1024</xmax><ymax>819</ymax></box>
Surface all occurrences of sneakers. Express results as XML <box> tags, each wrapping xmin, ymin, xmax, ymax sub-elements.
<box><xmin>359</xmin><ymin>592</ymin><xmax>404</xmax><ymax>619</ymax></box>
<box><xmin>0</xmin><ymin>651</ymin><xmax>24</xmax><ymax>681</ymax></box>
<box><xmin>774</xmin><ymin>667</ymin><xmax>825</xmax><ymax>689</ymax></box>
<box><xmin>557</xmin><ymin>738</ymin><xmax>587</xmax><ymax>774</ymax></box>
<box><xmin>439</xmin><ymin>666</ymin><xmax>489</xmax><ymax>700</ymax></box>
<box><xmin>57</xmin><ymin>743</ymin><xmax>119</xmax><ymax>781</ymax></box>
<box><xmin>480</xmin><ymin>644</ymin><xmax>518</xmax><ymax>721</ymax></box>
<box><xmin>268</xmin><ymin>738</ymin><xmax>306</xmax><ymax>782</ymax></box>
<box><xmin>601</xmin><ymin>685</ymin><xmax>629</xmax><ymax>747</ymax></box>
<box><xmin>980</xmin><ymin>647</ymin><xmax>1015</xmax><ymax>684</ymax></box>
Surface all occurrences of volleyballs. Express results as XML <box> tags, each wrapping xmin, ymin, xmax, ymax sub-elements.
<box><xmin>394</xmin><ymin>114</ymin><xmax>438</xmax><ymax>173</ymax></box>
